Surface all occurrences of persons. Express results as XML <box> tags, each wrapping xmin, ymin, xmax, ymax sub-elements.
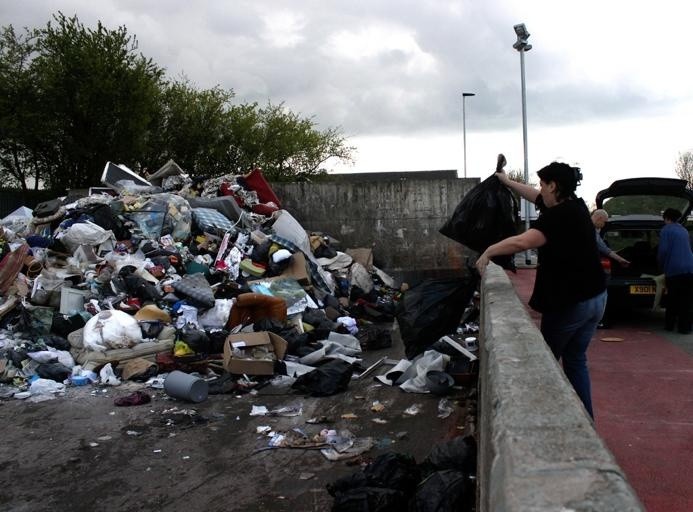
<box><xmin>657</xmin><ymin>208</ymin><xmax>693</xmax><ymax>334</ymax></box>
<box><xmin>590</xmin><ymin>209</ymin><xmax>630</xmax><ymax>327</ymax></box>
<box><xmin>476</xmin><ymin>162</ymin><xmax>608</xmax><ymax>421</ymax></box>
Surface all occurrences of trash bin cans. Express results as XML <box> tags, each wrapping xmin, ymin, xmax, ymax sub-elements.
<box><xmin>163</xmin><ymin>370</ymin><xmax>209</xmax><ymax>403</ymax></box>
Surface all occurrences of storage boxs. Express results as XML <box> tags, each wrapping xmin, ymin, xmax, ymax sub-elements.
<box><xmin>223</xmin><ymin>330</ymin><xmax>288</xmax><ymax>375</ymax></box>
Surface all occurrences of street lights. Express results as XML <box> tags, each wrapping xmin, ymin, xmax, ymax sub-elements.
<box><xmin>512</xmin><ymin>23</ymin><xmax>536</xmax><ymax>265</ymax></box>
<box><xmin>460</xmin><ymin>91</ymin><xmax>476</xmax><ymax>178</ymax></box>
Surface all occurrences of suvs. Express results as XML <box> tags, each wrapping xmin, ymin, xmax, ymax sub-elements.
<box><xmin>589</xmin><ymin>174</ymin><xmax>692</xmax><ymax>323</ymax></box>
